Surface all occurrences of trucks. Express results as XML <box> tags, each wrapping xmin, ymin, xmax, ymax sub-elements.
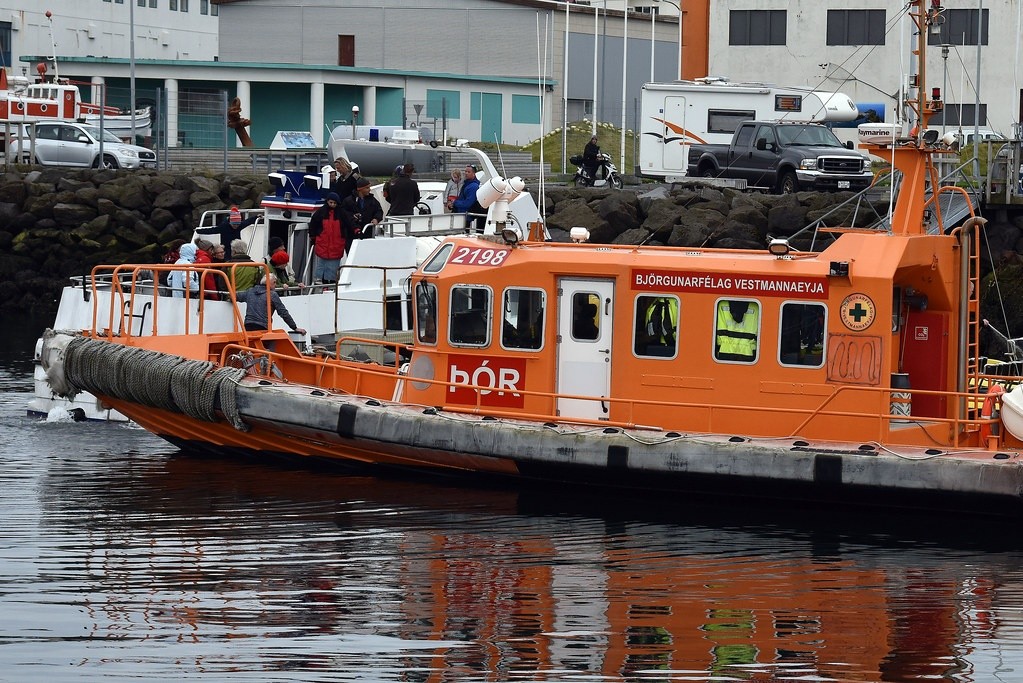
<box><xmin>639</xmin><ymin>76</ymin><xmax>888</xmax><ymax>178</ymax></box>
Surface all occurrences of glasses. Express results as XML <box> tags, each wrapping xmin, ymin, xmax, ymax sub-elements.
<box><xmin>591</xmin><ymin>138</ymin><xmax>597</xmax><ymax>140</ymax></box>
<box><xmin>467</xmin><ymin>163</ymin><xmax>476</xmax><ymax>169</ymax></box>
<box><xmin>215</xmin><ymin>251</ymin><xmax>225</xmax><ymax>255</ymax></box>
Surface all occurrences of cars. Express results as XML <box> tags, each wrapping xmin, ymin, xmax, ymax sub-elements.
<box><xmin>8</xmin><ymin>119</ymin><xmax>157</xmax><ymax>170</ymax></box>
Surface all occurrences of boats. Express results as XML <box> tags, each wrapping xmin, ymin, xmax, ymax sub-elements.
<box><xmin>0</xmin><ymin>11</ymin><xmax>153</xmax><ymax>152</ymax></box>
<box><xmin>24</xmin><ymin>9</ymin><xmax>553</xmax><ymax>423</ymax></box>
<box><xmin>37</xmin><ymin>0</ymin><xmax>1023</xmax><ymax>533</ymax></box>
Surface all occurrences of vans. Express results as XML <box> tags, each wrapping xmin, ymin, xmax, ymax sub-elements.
<box><xmin>953</xmin><ymin>130</ymin><xmax>1006</xmax><ymax>147</ymax></box>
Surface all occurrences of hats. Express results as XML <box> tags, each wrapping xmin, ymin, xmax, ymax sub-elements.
<box><xmin>356</xmin><ymin>176</ymin><xmax>372</xmax><ymax>191</ymax></box>
<box><xmin>268</xmin><ymin>237</ymin><xmax>284</xmax><ymax>251</ymax></box>
<box><xmin>194</xmin><ymin>238</ymin><xmax>213</xmax><ymax>251</ymax></box>
<box><xmin>271</xmin><ymin>249</ymin><xmax>289</xmax><ymax>265</ymax></box>
<box><xmin>229</xmin><ymin>207</ymin><xmax>242</xmax><ymax>225</ymax></box>
<box><xmin>395</xmin><ymin>165</ymin><xmax>405</xmax><ymax>177</ymax></box>
<box><xmin>321</xmin><ymin>164</ymin><xmax>336</xmax><ymax>173</ymax></box>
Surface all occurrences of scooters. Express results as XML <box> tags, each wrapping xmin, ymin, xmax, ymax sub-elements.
<box><xmin>570</xmin><ymin>150</ymin><xmax>624</xmax><ymax>190</ymax></box>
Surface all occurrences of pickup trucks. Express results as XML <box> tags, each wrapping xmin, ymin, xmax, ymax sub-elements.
<box><xmin>688</xmin><ymin>119</ymin><xmax>875</xmax><ymax>195</ymax></box>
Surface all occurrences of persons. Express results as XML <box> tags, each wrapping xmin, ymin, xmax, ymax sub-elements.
<box><xmin>334</xmin><ymin>157</ymin><xmax>362</xmax><ymax>201</ymax></box>
<box><xmin>223</xmin><ymin>272</ymin><xmax>307</xmax><ymax>337</ymax></box>
<box><xmin>383</xmin><ymin>165</ymin><xmax>404</xmax><ymax>198</ymax></box>
<box><xmin>259</xmin><ymin>235</ymin><xmax>305</xmax><ymax>296</ymax></box>
<box><xmin>218</xmin><ymin>238</ymin><xmax>259</xmax><ymax>300</ymax></box>
<box><xmin>132</xmin><ymin>238</ymin><xmax>225</xmax><ymax>300</ymax></box>
<box><xmin>308</xmin><ymin>192</ymin><xmax>361</xmax><ymax>291</ymax></box>
<box><xmin>192</xmin><ymin>207</ymin><xmax>263</xmax><ymax>260</ymax></box>
<box><xmin>447</xmin><ymin>163</ymin><xmax>483</xmax><ymax>232</ymax></box>
<box><xmin>443</xmin><ymin>168</ymin><xmax>464</xmax><ymax>207</ymax></box>
<box><xmin>583</xmin><ymin>135</ymin><xmax>600</xmax><ymax>180</ymax></box>
<box><xmin>340</xmin><ymin>178</ymin><xmax>383</xmax><ymax>257</ymax></box>
<box><xmin>383</xmin><ymin>161</ymin><xmax>420</xmax><ymax>228</ymax></box>
